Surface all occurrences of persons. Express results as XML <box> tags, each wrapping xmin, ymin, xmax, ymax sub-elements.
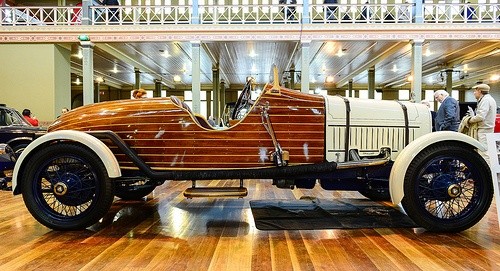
<box><xmin>207</xmin><ymin>116</ymin><xmax>218</xmax><ymax>127</ymax></box>
<box><xmin>278</xmin><ymin>0</ymin><xmax>476</xmax><ymax>25</ymax></box>
<box><xmin>57</xmin><ymin>107</ymin><xmax>69</xmax><ymax>119</ymax></box>
<box><xmin>68</xmin><ymin>0</ymin><xmax>121</xmax><ymax>26</ymax></box>
<box><xmin>420</xmin><ymin>89</ymin><xmax>460</xmax><ymax>132</ymax></box>
<box><xmin>22</xmin><ymin>108</ymin><xmax>39</xmax><ymax>127</ymax></box>
<box><xmin>461</xmin><ymin>83</ymin><xmax>497</xmax><ymax>168</ymax></box>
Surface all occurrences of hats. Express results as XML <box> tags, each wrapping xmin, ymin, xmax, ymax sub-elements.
<box><xmin>472</xmin><ymin>84</ymin><xmax>490</xmax><ymax>91</ymax></box>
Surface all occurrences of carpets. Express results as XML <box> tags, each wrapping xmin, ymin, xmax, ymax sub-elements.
<box><xmin>249</xmin><ymin>198</ymin><xmax>419</xmax><ymax>231</ymax></box>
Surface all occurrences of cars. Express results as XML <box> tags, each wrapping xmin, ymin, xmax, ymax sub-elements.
<box><xmin>11</xmin><ymin>64</ymin><xmax>494</xmax><ymax>235</ymax></box>
<box><xmin>0</xmin><ymin>104</ymin><xmax>49</xmax><ymax>168</ymax></box>
